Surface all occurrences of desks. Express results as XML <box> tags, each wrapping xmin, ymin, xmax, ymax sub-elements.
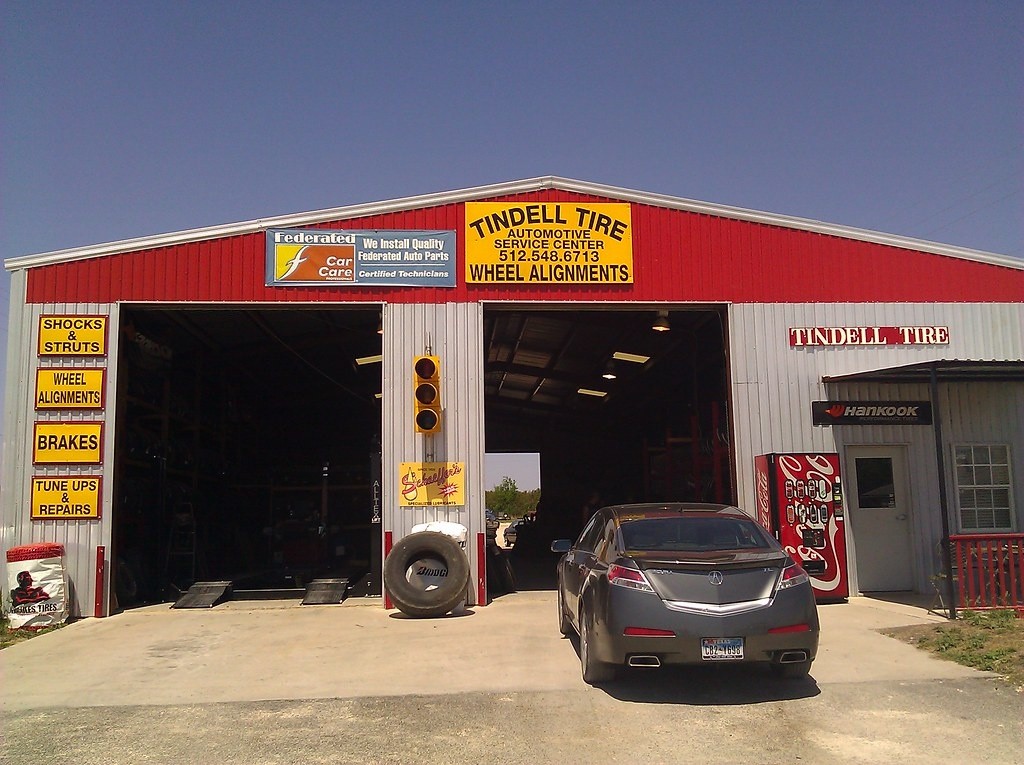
<box><xmin>970</xmin><ymin>548</ymin><xmax>1024</xmax><ymax>605</ymax></box>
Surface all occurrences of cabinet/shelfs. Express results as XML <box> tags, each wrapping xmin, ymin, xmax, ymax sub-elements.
<box><xmin>268</xmin><ymin>463</ymin><xmax>371</xmax><ymax>580</ymax></box>
<box><xmin>118</xmin><ymin>357</ymin><xmax>260</xmax><ymax>545</ymax></box>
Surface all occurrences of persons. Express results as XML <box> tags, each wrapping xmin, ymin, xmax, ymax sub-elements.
<box><xmin>582</xmin><ymin>493</ymin><xmax>600</xmax><ymax>528</ymax></box>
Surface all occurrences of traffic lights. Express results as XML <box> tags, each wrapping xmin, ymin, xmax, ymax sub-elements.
<box><xmin>411</xmin><ymin>355</ymin><xmax>441</xmax><ymax>433</ymax></box>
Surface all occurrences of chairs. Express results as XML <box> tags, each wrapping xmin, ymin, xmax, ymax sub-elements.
<box><xmin>927</xmin><ymin>533</ymin><xmax>976</xmax><ymax>618</ymax></box>
<box><xmin>687</xmin><ymin>524</ymin><xmax>721</xmax><ymax>546</ymax></box>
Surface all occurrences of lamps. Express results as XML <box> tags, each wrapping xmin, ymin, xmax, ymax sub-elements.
<box><xmin>377</xmin><ymin>312</ymin><xmax>382</xmax><ymax>335</ymax></box>
<box><xmin>600</xmin><ymin>358</ymin><xmax>617</xmax><ymax>380</ymax></box>
<box><xmin>650</xmin><ymin>310</ymin><xmax>672</xmax><ymax>333</ymax></box>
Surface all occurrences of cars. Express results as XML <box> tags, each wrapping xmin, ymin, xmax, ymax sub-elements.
<box><xmin>503</xmin><ymin>518</ymin><xmax>531</xmax><ymax>547</ymax></box>
<box><xmin>550</xmin><ymin>501</ymin><xmax>820</xmax><ymax>687</ymax></box>
